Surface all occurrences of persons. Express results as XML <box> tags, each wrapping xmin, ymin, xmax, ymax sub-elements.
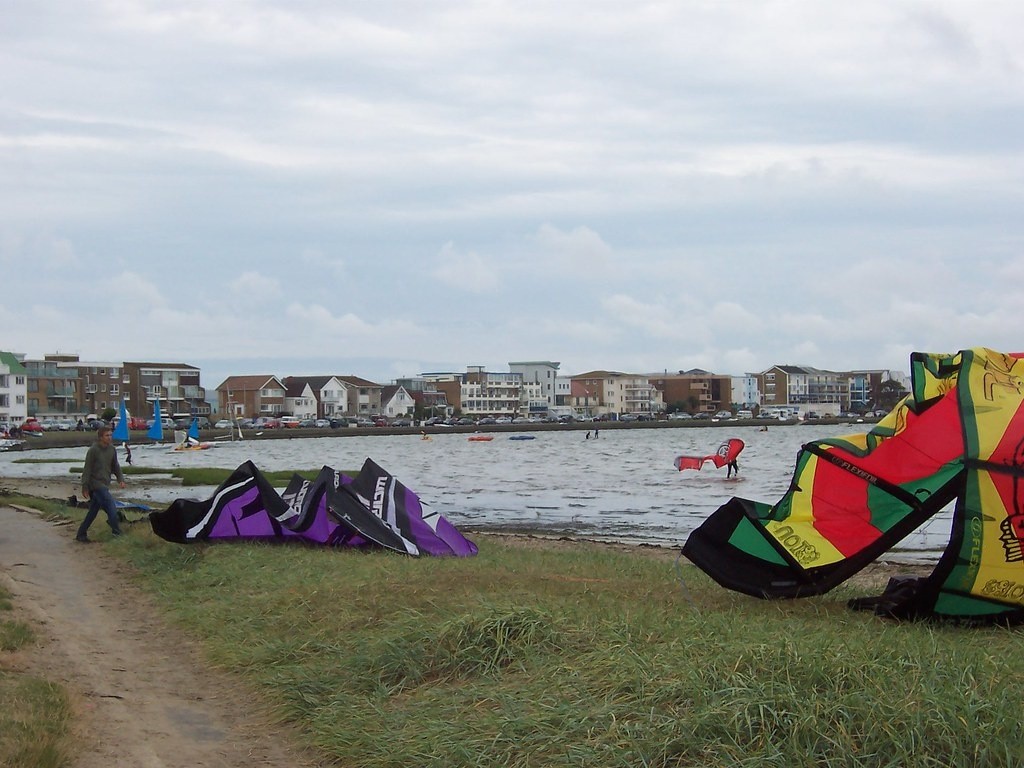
<box><xmin>727</xmin><ymin>459</ymin><xmax>738</xmax><ymax>478</ymax></box>
<box><xmin>586</xmin><ymin>431</ymin><xmax>590</xmax><ymax>439</ymax></box>
<box><xmin>76</xmin><ymin>428</ymin><xmax>125</xmax><ymax>543</ymax></box>
<box><xmin>595</xmin><ymin>426</ymin><xmax>599</xmax><ymax>438</ymax></box>
<box><xmin>124</xmin><ymin>445</ymin><xmax>134</xmax><ymax>465</ymax></box>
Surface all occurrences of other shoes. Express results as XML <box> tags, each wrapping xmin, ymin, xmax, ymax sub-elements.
<box><xmin>111</xmin><ymin>526</ymin><xmax>127</xmax><ymax>536</ymax></box>
<box><xmin>75</xmin><ymin>536</ymin><xmax>93</xmax><ymax>545</ymax></box>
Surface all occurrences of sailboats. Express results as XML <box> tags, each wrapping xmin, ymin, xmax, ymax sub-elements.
<box><xmin>142</xmin><ymin>399</ymin><xmax>172</xmax><ymax>449</ymax></box>
<box><xmin>112</xmin><ymin>397</ymin><xmax>138</xmax><ymax>451</ymax></box>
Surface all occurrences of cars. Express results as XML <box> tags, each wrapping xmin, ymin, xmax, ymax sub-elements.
<box><xmin>792</xmin><ymin>411</ymin><xmax>860</xmax><ymax>419</ymax></box>
<box><xmin>299</xmin><ymin>417</ymin><xmax>389</xmax><ymax>428</ymax></box>
<box><xmin>620</xmin><ymin>414</ymin><xmax>636</xmax><ymax>422</ymax></box>
<box><xmin>669</xmin><ymin>413</ymin><xmax>710</xmax><ymax>421</ymax></box>
<box><xmin>214</xmin><ymin>420</ymin><xmax>235</xmax><ymax>429</ymax></box>
<box><xmin>127</xmin><ymin>417</ymin><xmax>155</xmax><ymax>431</ymax></box>
<box><xmin>512</xmin><ymin>413</ymin><xmax>610</xmax><ymax>423</ymax></box>
<box><xmin>715</xmin><ymin>411</ymin><xmax>732</xmax><ymax>419</ymax></box>
<box><xmin>637</xmin><ymin>414</ymin><xmax>653</xmax><ymax>422</ymax></box>
<box><xmin>865</xmin><ymin>410</ymin><xmax>888</xmax><ymax>418</ymax></box>
<box><xmin>392</xmin><ymin>420</ymin><xmax>411</xmax><ymax>428</ymax></box>
<box><xmin>424</xmin><ymin>417</ymin><xmax>511</xmax><ymax>426</ymax></box>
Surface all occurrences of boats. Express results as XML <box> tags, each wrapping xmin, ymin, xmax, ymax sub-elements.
<box><xmin>423</xmin><ymin>433</ymin><xmax>433</xmax><ymax>441</ymax></box>
<box><xmin>510</xmin><ymin>436</ymin><xmax>536</xmax><ymax>440</ymax></box>
<box><xmin>469</xmin><ymin>436</ymin><xmax>495</xmax><ymax>441</ymax></box>
<box><xmin>171</xmin><ymin>441</ymin><xmax>213</xmax><ymax>451</ymax></box>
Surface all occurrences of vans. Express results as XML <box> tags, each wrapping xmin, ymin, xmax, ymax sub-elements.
<box><xmin>22</xmin><ymin>418</ymin><xmax>78</xmax><ymax>432</ymax></box>
<box><xmin>236</xmin><ymin>416</ymin><xmax>298</xmax><ymax>429</ymax></box>
<box><xmin>736</xmin><ymin>411</ymin><xmax>754</xmax><ymax>419</ymax></box>
<box><xmin>756</xmin><ymin>410</ymin><xmax>787</xmax><ymax>419</ymax></box>
<box><xmin>163</xmin><ymin>417</ymin><xmax>211</xmax><ymax>429</ymax></box>
